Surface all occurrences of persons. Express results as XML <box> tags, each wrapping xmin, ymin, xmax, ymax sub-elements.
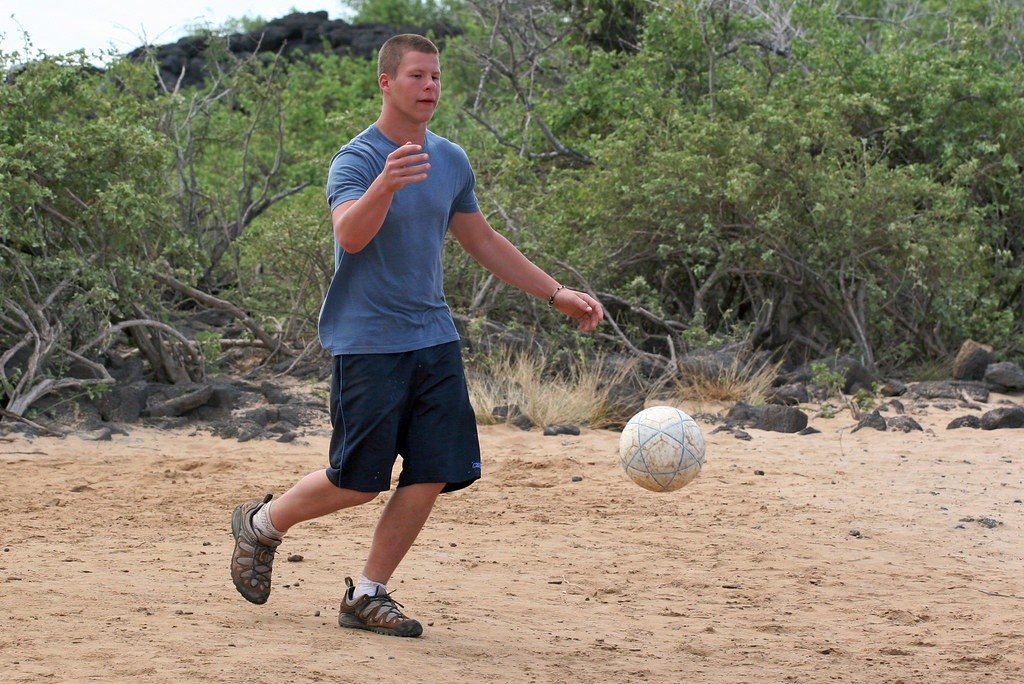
<box><xmin>231</xmin><ymin>35</ymin><xmax>604</xmax><ymax>638</ymax></box>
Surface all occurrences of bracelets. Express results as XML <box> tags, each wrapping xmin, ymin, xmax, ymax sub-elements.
<box><xmin>548</xmin><ymin>285</ymin><xmax>567</xmax><ymax>309</ymax></box>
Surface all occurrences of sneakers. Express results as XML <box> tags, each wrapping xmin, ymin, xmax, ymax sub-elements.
<box><xmin>338</xmin><ymin>576</ymin><xmax>423</xmax><ymax>637</ymax></box>
<box><xmin>230</xmin><ymin>494</ymin><xmax>282</xmax><ymax>605</ymax></box>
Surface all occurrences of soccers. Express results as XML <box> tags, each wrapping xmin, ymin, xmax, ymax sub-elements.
<box><xmin>619</xmin><ymin>405</ymin><xmax>706</xmax><ymax>492</ymax></box>
